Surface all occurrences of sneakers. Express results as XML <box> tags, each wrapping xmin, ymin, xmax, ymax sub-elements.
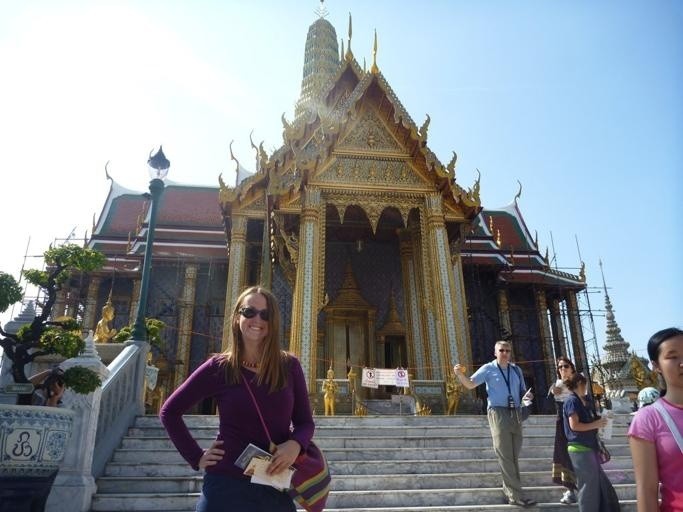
<box><xmin>560</xmin><ymin>489</ymin><xmax>576</xmax><ymax>503</ymax></box>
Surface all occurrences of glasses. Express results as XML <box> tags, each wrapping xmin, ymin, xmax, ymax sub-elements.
<box><xmin>237</xmin><ymin>307</ymin><xmax>270</xmax><ymax>320</ymax></box>
<box><xmin>499</xmin><ymin>350</ymin><xmax>510</xmax><ymax>352</ymax></box>
<box><xmin>557</xmin><ymin>365</ymin><xmax>569</xmax><ymax>368</ymax></box>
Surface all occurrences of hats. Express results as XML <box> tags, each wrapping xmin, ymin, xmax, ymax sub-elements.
<box><xmin>638</xmin><ymin>387</ymin><xmax>661</xmax><ymax>407</ymax></box>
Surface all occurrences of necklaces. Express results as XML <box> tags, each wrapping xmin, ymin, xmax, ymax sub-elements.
<box><xmin>241</xmin><ymin>360</ymin><xmax>259</xmax><ymax>368</ymax></box>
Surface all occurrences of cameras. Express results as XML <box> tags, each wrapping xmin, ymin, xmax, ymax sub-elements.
<box><xmin>57</xmin><ymin>375</ymin><xmax>65</xmax><ymax>384</ymax></box>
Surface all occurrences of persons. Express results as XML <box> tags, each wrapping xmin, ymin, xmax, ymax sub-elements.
<box><xmin>546</xmin><ymin>356</ymin><xmax>579</xmax><ymax>505</ymax></box>
<box><xmin>454</xmin><ymin>340</ymin><xmax>537</xmax><ymax>506</ymax></box>
<box><xmin>322</xmin><ymin>370</ymin><xmax>338</xmax><ymax>416</ymax></box>
<box><xmin>446</xmin><ymin>371</ymin><xmax>463</xmax><ymax>416</ymax></box>
<box><xmin>159</xmin><ymin>285</ymin><xmax>314</xmax><ymax>512</ymax></box>
<box><xmin>626</xmin><ymin>327</ymin><xmax>683</xmax><ymax>512</ymax></box>
<box><xmin>94</xmin><ymin>305</ymin><xmax>116</xmax><ymax>343</ymax></box>
<box><xmin>562</xmin><ymin>372</ymin><xmax>621</xmax><ymax>512</ymax></box>
<box><xmin>27</xmin><ymin>367</ymin><xmax>75</xmax><ymax>410</ymax></box>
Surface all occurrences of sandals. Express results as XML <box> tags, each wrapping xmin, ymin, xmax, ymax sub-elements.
<box><xmin>503</xmin><ymin>493</ymin><xmax>536</xmax><ymax>506</ymax></box>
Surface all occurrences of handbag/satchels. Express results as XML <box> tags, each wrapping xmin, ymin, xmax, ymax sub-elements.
<box><xmin>269</xmin><ymin>432</ymin><xmax>331</xmax><ymax>512</ymax></box>
<box><xmin>594</xmin><ymin>433</ymin><xmax>610</xmax><ymax>464</ymax></box>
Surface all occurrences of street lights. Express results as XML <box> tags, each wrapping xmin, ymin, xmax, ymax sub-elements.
<box><xmin>130</xmin><ymin>146</ymin><xmax>170</xmax><ymax>340</ymax></box>
